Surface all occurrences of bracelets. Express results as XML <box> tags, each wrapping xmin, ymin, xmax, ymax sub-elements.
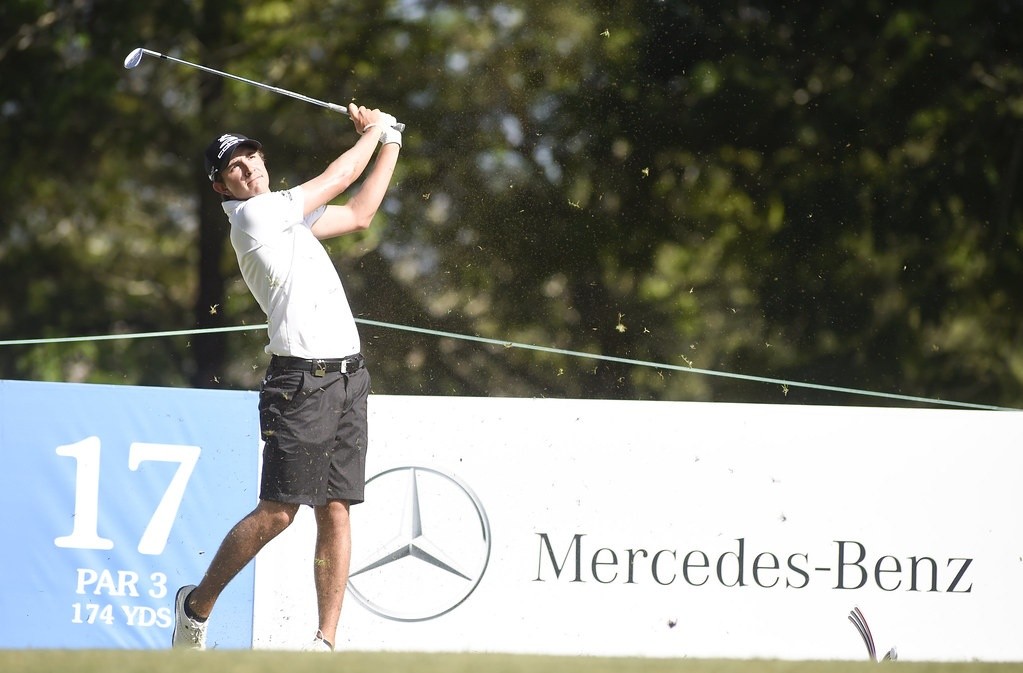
<box><xmin>362</xmin><ymin>124</ymin><xmax>380</xmax><ymax>135</ymax></box>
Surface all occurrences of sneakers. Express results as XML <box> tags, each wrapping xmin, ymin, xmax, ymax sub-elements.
<box><xmin>302</xmin><ymin>628</ymin><xmax>330</xmax><ymax>652</ymax></box>
<box><xmin>172</xmin><ymin>585</ymin><xmax>209</xmax><ymax>649</ymax></box>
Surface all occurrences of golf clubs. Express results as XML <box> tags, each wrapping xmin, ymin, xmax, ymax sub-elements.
<box><xmin>122</xmin><ymin>47</ymin><xmax>407</xmax><ymax>134</ymax></box>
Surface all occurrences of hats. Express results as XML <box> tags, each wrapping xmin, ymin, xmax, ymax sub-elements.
<box><xmin>204</xmin><ymin>133</ymin><xmax>262</xmax><ymax>181</ymax></box>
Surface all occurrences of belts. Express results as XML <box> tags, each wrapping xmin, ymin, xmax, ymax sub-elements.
<box><xmin>271</xmin><ymin>356</ymin><xmax>364</xmax><ymax>374</ymax></box>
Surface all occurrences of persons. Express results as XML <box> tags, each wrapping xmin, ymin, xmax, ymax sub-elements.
<box><xmin>172</xmin><ymin>103</ymin><xmax>401</xmax><ymax>651</ymax></box>
